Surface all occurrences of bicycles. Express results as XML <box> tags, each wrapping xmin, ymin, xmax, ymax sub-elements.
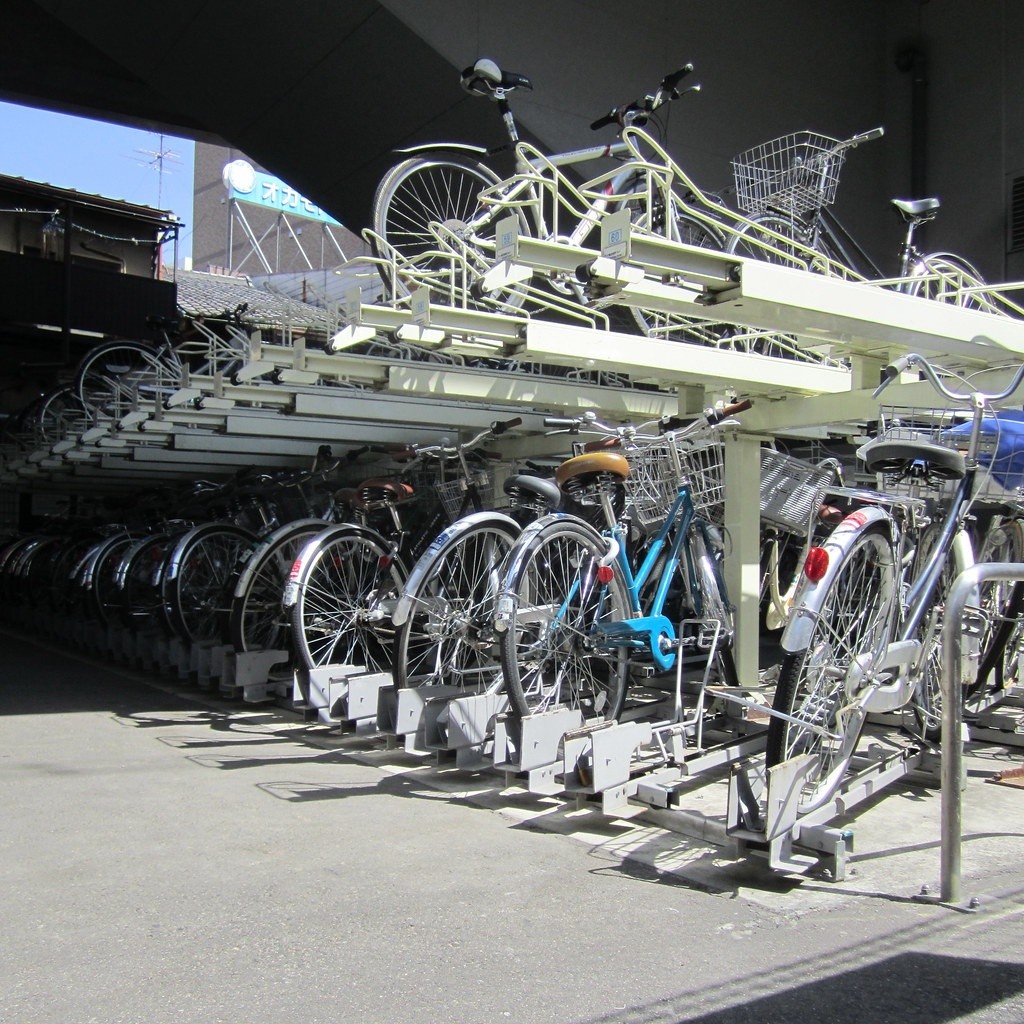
<box><xmin>30</xmin><ymin>301</ymin><xmax>281</xmax><ymax>441</ymax></box>
<box><xmin>0</xmin><ymin>355</ymin><xmax>1024</xmax><ymax>818</ymax></box>
<box><xmin>722</xmin><ymin>126</ymin><xmax>995</xmax><ymax>379</ymax></box>
<box><xmin>368</xmin><ymin>58</ymin><xmax>730</xmax><ymax>339</ymax></box>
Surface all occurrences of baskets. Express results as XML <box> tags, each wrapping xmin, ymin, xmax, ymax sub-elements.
<box><xmin>673</xmin><ymin>182</ymin><xmax>727</xmax><ymax>249</ymax></box>
<box><xmin>877</xmin><ymin>403</ymin><xmax>1024</xmax><ymax>504</ymax></box>
<box><xmin>756</xmin><ymin>445</ymin><xmax>834</xmax><ymax>537</ymax></box>
<box><xmin>729</xmin><ymin>131</ymin><xmax>847</xmax><ymax>214</ymax></box>
<box><xmin>288</xmin><ymin>459</ymin><xmax>525</xmax><ymax>519</ymax></box>
<box><xmin>566</xmin><ymin>435</ymin><xmax>730</xmax><ymax>524</ymax></box>
